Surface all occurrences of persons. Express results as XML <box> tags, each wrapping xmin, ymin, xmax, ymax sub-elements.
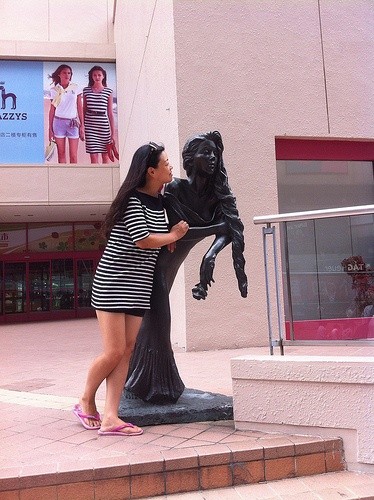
<box><xmin>73</xmin><ymin>141</ymin><xmax>188</xmax><ymax>436</ymax></box>
<box><xmin>43</xmin><ymin>64</ymin><xmax>119</xmax><ymax>164</ymax></box>
<box><xmin>125</xmin><ymin>131</ymin><xmax>247</xmax><ymax>403</ymax></box>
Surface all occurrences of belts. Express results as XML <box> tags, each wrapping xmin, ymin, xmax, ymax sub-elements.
<box><xmin>88</xmin><ymin>110</ymin><xmax>105</xmax><ymax>114</ymax></box>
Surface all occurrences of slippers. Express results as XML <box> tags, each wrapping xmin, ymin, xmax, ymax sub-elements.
<box><xmin>97</xmin><ymin>423</ymin><xmax>143</xmax><ymax>436</ymax></box>
<box><xmin>74</xmin><ymin>404</ymin><xmax>102</xmax><ymax>429</ymax></box>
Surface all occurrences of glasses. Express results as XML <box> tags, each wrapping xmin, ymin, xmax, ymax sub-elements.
<box><xmin>147</xmin><ymin>141</ymin><xmax>159</xmax><ymax>168</ymax></box>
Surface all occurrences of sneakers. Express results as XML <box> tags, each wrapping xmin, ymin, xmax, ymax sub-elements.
<box><xmin>46</xmin><ymin>140</ymin><xmax>56</xmax><ymax>161</ymax></box>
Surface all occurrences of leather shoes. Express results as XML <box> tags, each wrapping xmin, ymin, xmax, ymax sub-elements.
<box><xmin>106</xmin><ymin>143</ymin><xmax>119</xmax><ymax>162</ymax></box>
